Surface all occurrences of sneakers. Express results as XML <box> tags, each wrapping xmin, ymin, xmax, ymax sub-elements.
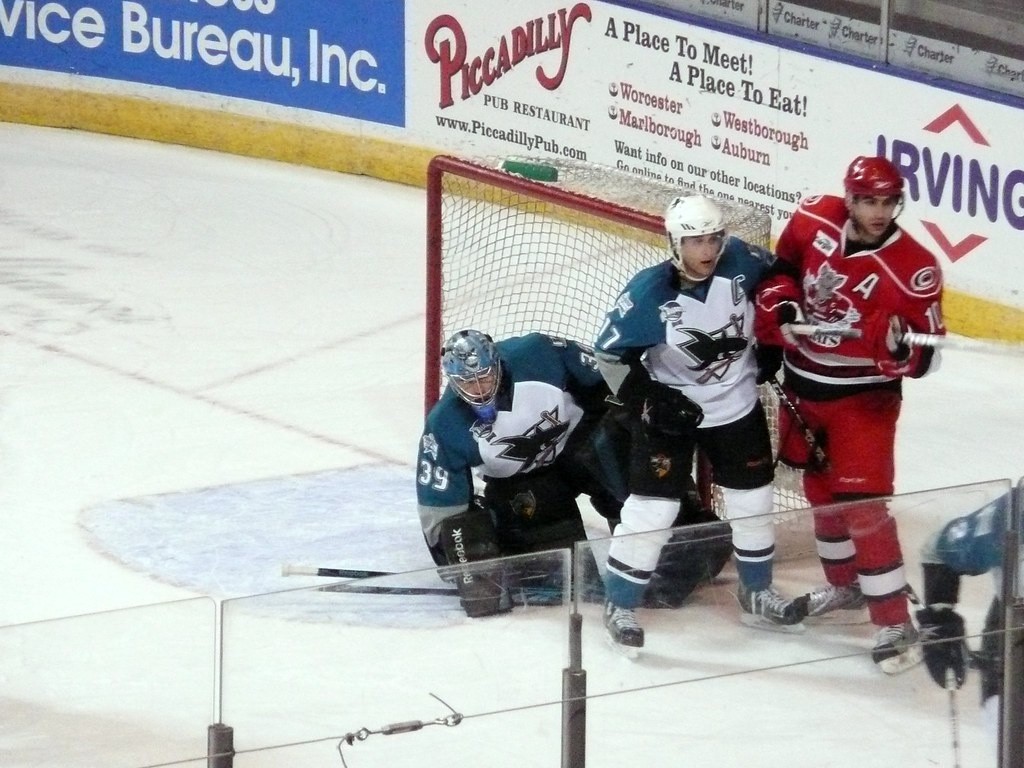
<box><xmin>737</xmin><ymin>585</ymin><xmax>806</xmax><ymax>634</ymax></box>
<box><xmin>870</xmin><ymin>614</ymin><xmax>925</xmax><ymax>674</ymax></box>
<box><xmin>602</xmin><ymin>598</ymin><xmax>644</xmax><ymax>659</ymax></box>
<box><xmin>803</xmin><ymin>583</ymin><xmax>872</xmax><ymax>625</ymax></box>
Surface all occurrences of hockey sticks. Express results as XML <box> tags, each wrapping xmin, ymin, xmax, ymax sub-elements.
<box><xmin>944</xmin><ymin>659</ymin><xmax>963</xmax><ymax>768</ymax></box>
<box><xmin>324</xmin><ymin>584</ymin><xmax>464</xmax><ymax>597</ymax></box>
<box><xmin>282</xmin><ymin>566</ymin><xmax>389</xmax><ymax>579</ymax></box>
<box><xmin>785</xmin><ymin>323</ymin><xmax>1023</xmax><ymax>362</ymax></box>
<box><xmin>743</xmin><ymin>341</ymin><xmax>927</xmax><ymax>613</ymax></box>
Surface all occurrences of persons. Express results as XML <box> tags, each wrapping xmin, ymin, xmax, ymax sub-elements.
<box><xmin>758</xmin><ymin>156</ymin><xmax>946</xmax><ymax>674</ymax></box>
<box><xmin>594</xmin><ymin>196</ymin><xmax>807</xmax><ymax>658</ymax></box>
<box><xmin>923</xmin><ymin>486</ymin><xmax>1024</xmax><ymax>730</ymax></box>
<box><xmin>415</xmin><ymin>330</ymin><xmax>734</xmax><ymax>619</ymax></box>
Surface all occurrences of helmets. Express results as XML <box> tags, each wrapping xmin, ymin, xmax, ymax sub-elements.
<box><xmin>844</xmin><ymin>156</ymin><xmax>903</xmax><ymax>195</ymax></box>
<box><xmin>665</xmin><ymin>195</ymin><xmax>727</xmax><ymax>282</ymax></box>
<box><xmin>441</xmin><ymin>330</ymin><xmax>501</xmax><ymax>424</ymax></box>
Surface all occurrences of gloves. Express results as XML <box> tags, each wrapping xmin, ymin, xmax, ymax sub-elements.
<box><xmin>873</xmin><ymin>311</ymin><xmax>934</xmax><ymax>379</ymax></box>
<box><xmin>754</xmin><ymin>275</ymin><xmax>806</xmax><ymax>353</ymax></box>
<box><xmin>917</xmin><ymin>605</ymin><xmax>966</xmax><ymax>691</ymax></box>
<box><xmin>616</xmin><ymin>364</ymin><xmax>703</xmax><ymax>432</ymax></box>
<box><xmin>755</xmin><ymin>345</ymin><xmax>784</xmax><ymax>386</ymax></box>
